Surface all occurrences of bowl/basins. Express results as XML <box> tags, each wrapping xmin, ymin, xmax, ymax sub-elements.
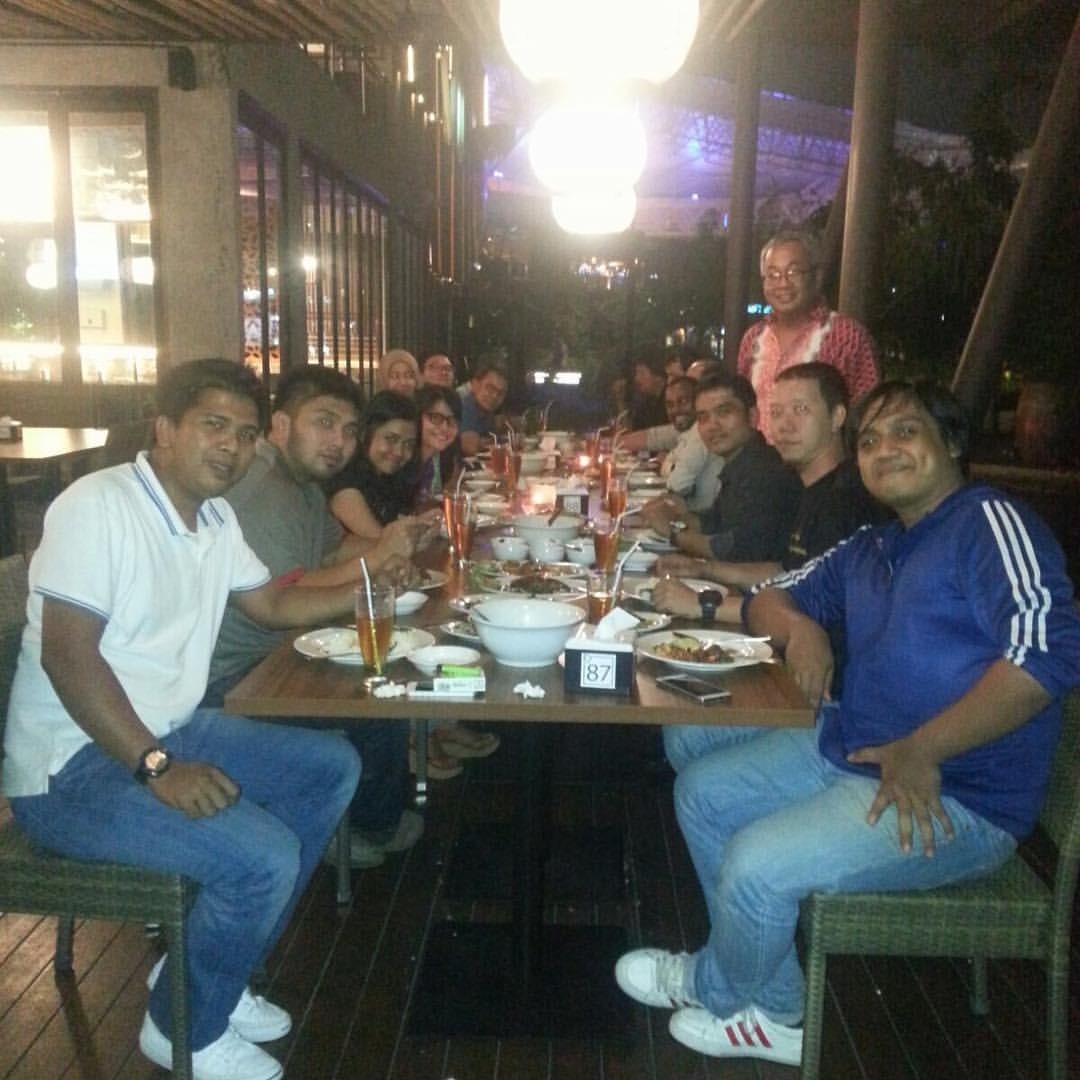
<box><xmin>407</xmin><ymin>646</ymin><xmax>481</xmax><ymax>677</ymax></box>
<box><xmin>464</xmin><ymin>430</ymin><xmax>668</xmax><ymax>515</ymax></box>
<box><xmin>490</xmin><ymin>536</ymin><xmax>529</xmax><ymax>561</ymax></box>
<box><xmin>510</xmin><ymin>514</ymin><xmax>584</xmax><ymax>543</ymax></box>
<box><xmin>565</xmin><ymin>538</ymin><xmax>596</xmax><ymax>566</ymax></box>
<box><xmin>469</xmin><ymin>599</ymin><xmax>586</xmax><ymax>668</ymax></box>
<box><xmin>615</xmin><ymin>551</ymin><xmax>660</xmax><ymax>572</ymax></box>
<box><xmin>527</xmin><ymin>537</ymin><xmax>565</xmax><ymax>561</ymax></box>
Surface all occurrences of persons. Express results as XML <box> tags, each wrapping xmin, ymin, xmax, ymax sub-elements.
<box><xmin>617</xmin><ymin>382</ymin><xmax>1080</xmax><ymax>1065</ymax></box>
<box><xmin>209</xmin><ymin>342</ymin><xmax>899</xmax><ymax>865</ymax></box>
<box><xmin>734</xmin><ymin>231</ymin><xmax>884</xmax><ymax>445</ymax></box>
<box><xmin>0</xmin><ymin>350</ymin><xmax>423</xmax><ymax>1080</ymax></box>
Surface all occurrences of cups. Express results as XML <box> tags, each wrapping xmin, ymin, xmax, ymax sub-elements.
<box><xmin>442</xmin><ymin>492</ymin><xmax>473</xmax><ymax>569</ymax></box>
<box><xmin>599</xmin><ymin>460</ymin><xmax>618</xmax><ymax>512</ymax></box>
<box><xmin>588</xmin><ymin>568</ymin><xmax>624</xmax><ymax>625</ymax></box>
<box><xmin>507</xmin><ymin>455</ymin><xmax>521</xmax><ymax>489</ymax></box>
<box><xmin>593</xmin><ymin>517</ymin><xmax>621</xmax><ymax>577</ymax></box>
<box><xmin>494</xmin><ymin>471</ymin><xmax>516</xmax><ymax>503</ymax></box>
<box><xmin>492</xmin><ymin>443</ymin><xmax>507</xmax><ymax>473</ymax></box>
<box><xmin>355</xmin><ymin>583</ymin><xmax>395</xmax><ymax>689</ymax></box>
<box><xmin>609</xmin><ymin>478</ymin><xmax>627</xmax><ymax>518</ymax></box>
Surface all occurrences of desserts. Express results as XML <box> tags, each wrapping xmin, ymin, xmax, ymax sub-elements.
<box><xmin>669</xmin><ymin>637</ymin><xmax>703</xmax><ymax>661</ymax></box>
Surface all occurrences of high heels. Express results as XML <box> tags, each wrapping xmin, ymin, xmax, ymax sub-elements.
<box><xmin>405</xmin><ymin>733</ymin><xmax>462</xmax><ymax>779</ymax></box>
<box><xmin>412</xmin><ymin>723</ymin><xmax>499</xmax><ymax>759</ymax></box>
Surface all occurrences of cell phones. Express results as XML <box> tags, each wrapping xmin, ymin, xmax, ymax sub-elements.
<box><xmin>406</xmin><ymin>682</ymin><xmax>482</xmax><ymax>697</ymax></box>
<box><xmin>658</xmin><ymin>673</ymin><xmax>732</xmax><ymax>701</ymax></box>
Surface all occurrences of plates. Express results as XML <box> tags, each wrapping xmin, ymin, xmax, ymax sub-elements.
<box><xmin>650</xmin><ymin>575</ymin><xmax>729</xmax><ymax>600</ymax></box>
<box><xmin>396</xmin><ymin>568</ymin><xmax>449</xmax><ymax>590</ymax></box>
<box><xmin>636</xmin><ymin>629</ymin><xmax>774</xmax><ymax>672</ymax></box>
<box><xmin>293</xmin><ymin>623</ymin><xmax>437</xmax><ymax>665</ymax></box>
<box><xmin>392</xmin><ymin>591</ymin><xmax>430</xmax><ymax>616</ymax></box>
<box><xmin>439</xmin><ymin>620</ymin><xmax>480</xmax><ymax>642</ymax></box>
<box><xmin>476</xmin><ymin>561</ymin><xmax>588</xmax><ymax>600</ymax></box>
<box><xmin>640</xmin><ymin>528</ymin><xmax>680</xmax><ymax>552</ymax></box>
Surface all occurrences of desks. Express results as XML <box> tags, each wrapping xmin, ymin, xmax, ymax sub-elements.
<box><xmin>0</xmin><ymin>427</ymin><xmax>107</xmax><ymax>559</ymax></box>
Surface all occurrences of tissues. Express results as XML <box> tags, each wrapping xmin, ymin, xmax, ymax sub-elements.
<box><xmin>563</xmin><ymin>604</ymin><xmax>642</xmax><ymax>697</ymax></box>
<box><xmin>537</xmin><ymin>436</ymin><xmax>561</xmax><ymax>470</ymax></box>
<box><xmin>557</xmin><ymin>473</ymin><xmax>590</xmax><ymax>517</ymax></box>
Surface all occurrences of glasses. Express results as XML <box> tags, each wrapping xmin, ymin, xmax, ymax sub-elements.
<box><xmin>762</xmin><ymin>268</ymin><xmax>809</xmax><ymax>282</ymax></box>
<box><xmin>424</xmin><ymin>411</ymin><xmax>458</xmax><ymax>426</ymax></box>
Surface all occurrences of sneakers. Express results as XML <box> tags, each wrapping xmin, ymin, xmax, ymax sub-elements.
<box><xmin>137</xmin><ymin>1008</ymin><xmax>285</xmax><ymax>1080</ymax></box>
<box><xmin>667</xmin><ymin>1001</ymin><xmax>802</xmax><ymax>1065</ymax></box>
<box><xmin>145</xmin><ymin>954</ymin><xmax>290</xmax><ymax>1044</ymax></box>
<box><xmin>614</xmin><ymin>948</ymin><xmax>709</xmax><ymax>1010</ymax></box>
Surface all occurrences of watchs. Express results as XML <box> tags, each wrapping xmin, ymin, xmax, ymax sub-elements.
<box><xmin>669</xmin><ymin>519</ymin><xmax>689</xmax><ymax>547</ymax></box>
<box><xmin>699</xmin><ymin>588</ymin><xmax>723</xmax><ymax>623</ymax></box>
<box><xmin>135</xmin><ymin>746</ymin><xmax>170</xmax><ymax>787</ymax></box>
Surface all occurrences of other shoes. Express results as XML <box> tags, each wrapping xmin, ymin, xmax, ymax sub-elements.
<box><xmin>380</xmin><ymin>811</ymin><xmax>423</xmax><ymax>851</ymax></box>
<box><xmin>318</xmin><ymin>830</ymin><xmax>384</xmax><ymax>869</ymax></box>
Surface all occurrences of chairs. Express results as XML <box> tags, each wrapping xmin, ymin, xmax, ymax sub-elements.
<box><xmin>799</xmin><ymin>692</ymin><xmax>1080</xmax><ymax>1080</ymax></box>
<box><xmin>0</xmin><ymin>624</ymin><xmax>353</xmax><ymax>1080</ymax></box>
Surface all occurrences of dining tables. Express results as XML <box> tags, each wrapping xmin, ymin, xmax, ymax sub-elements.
<box><xmin>226</xmin><ymin>430</ymin><xmax>813</xmax><ymax>1036</ymax></box>
